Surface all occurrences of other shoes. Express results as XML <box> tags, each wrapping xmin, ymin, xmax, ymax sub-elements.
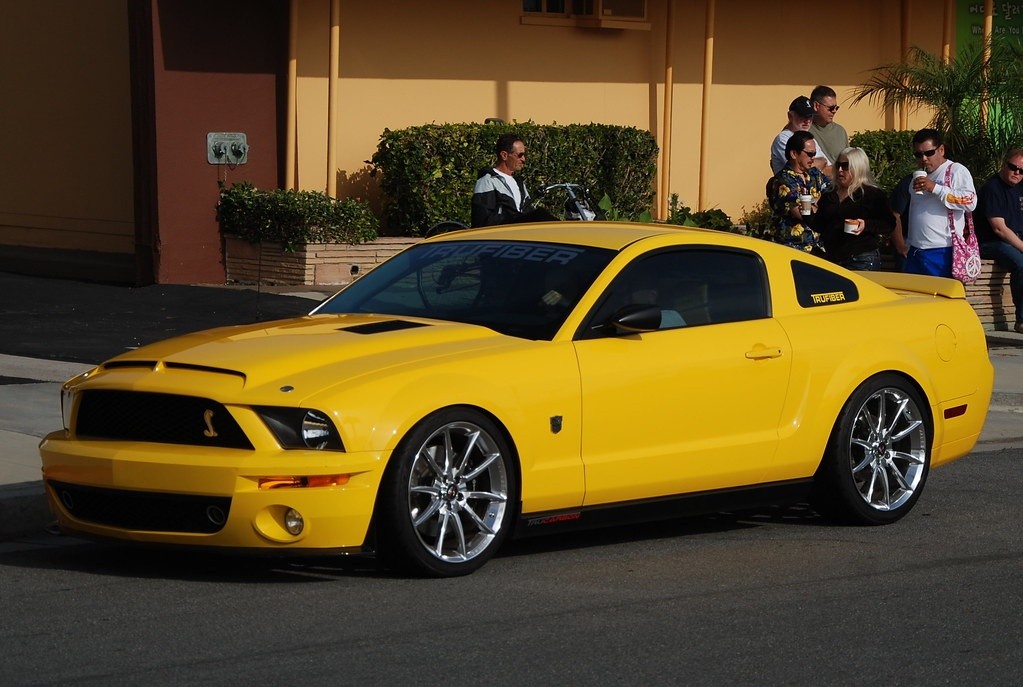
<box><xmin>1014</xmin><ymin>321</ymin><xmax>1023</xmax><ymax>334</ymax></box>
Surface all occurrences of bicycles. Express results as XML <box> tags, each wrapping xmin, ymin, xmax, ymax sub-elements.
<box><xmin>417</xmin><ymin>183</ymin><xmax>608</xmax><ymax>311</ymax></box>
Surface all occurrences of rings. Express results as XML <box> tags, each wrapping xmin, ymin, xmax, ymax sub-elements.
<box><xmin>921</xmin><ymin>183</ymin><xmax>924</xmax><ymax>186</ymax></box>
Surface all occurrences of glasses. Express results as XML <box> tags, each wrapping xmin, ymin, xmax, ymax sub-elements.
<box><xmin>818</xmin><ymin>101</ymin><xmax>839</xmax><ymax>111</ymax></box>
<box><xmin>836</xmin><ymin>160</ymin><xmax>849</xmax><ymax>171</ymax></box>
<box><xmin>515</xmin><ymin>151</ymin><xmax>526</xmax><ymax>158</ymax></box>
<box><xmin>913</xmin><ymin>145</ymin><xmax>939</xmax><ymax>158</ymax></box>
<box><xmin>802</xmin><ymin>149</ymin><xmax>816</xmax><ymax>157</ymax></box>
<box><xmin>1006</xmin><ymin>162</ymin><xmax>1023</xmax><ymax>175</ymax></box>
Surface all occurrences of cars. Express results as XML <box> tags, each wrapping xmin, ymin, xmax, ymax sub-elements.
<box><xmin>38</xmin><ymin>218</ymin><xmax>995</xmax><ymax>579</ymax></box>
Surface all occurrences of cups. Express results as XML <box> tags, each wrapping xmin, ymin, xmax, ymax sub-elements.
<box><xmin>914</xmin><ymin>171</ymin><xmax>927</xmax><ymax>195</ymax></box>
<box><xmin>801</xmin><ymin>195</ymin><xmax>812</xmax><ymax>216</ymax></box>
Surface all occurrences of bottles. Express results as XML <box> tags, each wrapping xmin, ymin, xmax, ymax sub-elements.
<box><xmin>844</xmin><ymin>219</ymin><xmax>860</xmax><ymax>233</ymax></box>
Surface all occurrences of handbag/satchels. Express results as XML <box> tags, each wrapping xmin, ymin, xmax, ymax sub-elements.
<box><xmin>952</xmin><ymin>233</ymin><xmax>981</xmax><ymax>283</ymax></box>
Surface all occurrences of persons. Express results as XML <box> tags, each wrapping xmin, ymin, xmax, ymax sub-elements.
<box><xmin>887</xmin><ymin>168</ymin><xmax>914</xmax><ymax>273</ymax></box>
<box><xmin>973</xmin><ymin>146</ymin><xmax>1023</xmax><ymax>333</ymax></box>
<box><xmin>796</xmin><ymin>147</ymin><xmax>896</xmax><ymax>272</ymax></box>
<box><xmin>771</xmin><ymin>95</ymin><xmax>835</xmax><ymax>175</ymax></box>
<box><xmin>900</xmin><ymin>129</ymin><xmax>977</xmax><ymax>278</ymax></box>
<box><xmin>471</xmin><ymin>133</ymin><xmax>560</xmax><ymax>228</ymax></box>
<box><xmin>783</xmin><ymin>85</ymin><xmax>850</xmax><ymax>171</ymax></box>
<box><xmin>765</xmin><ymin>130</ymin><xmax>836</xmax><ymax>262</ymax></box>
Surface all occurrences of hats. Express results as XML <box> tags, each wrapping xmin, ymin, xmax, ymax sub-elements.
<box><xmin>789</xmin><ymin>96</ymin><xmax>814</xmax><ymax>117</ymax></box>
